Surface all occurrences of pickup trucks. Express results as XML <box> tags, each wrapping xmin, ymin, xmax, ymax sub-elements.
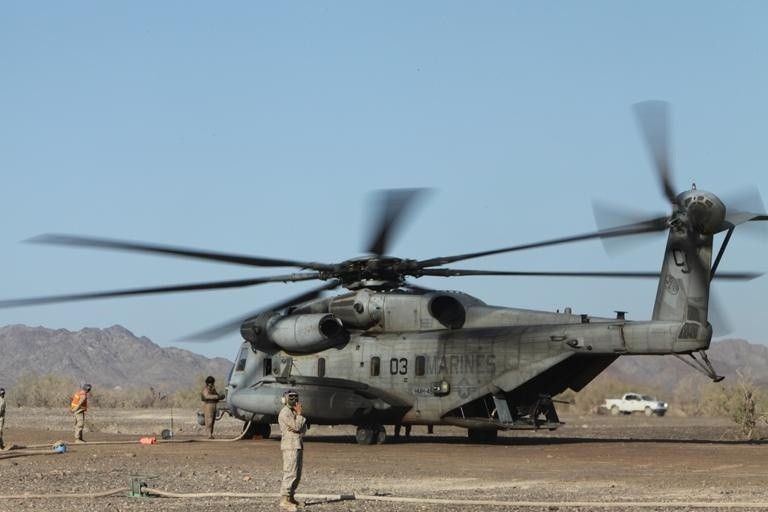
<box><xmin>603</xmin><ymin>393</ymin><xmax>668</xmax><ymax>416</ymax></box>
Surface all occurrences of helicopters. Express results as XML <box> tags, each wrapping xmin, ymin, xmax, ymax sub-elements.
<box><xmin>0</xmin><ymin>99</ymin><xmax>768</xmax><ymax>443</ymax></box>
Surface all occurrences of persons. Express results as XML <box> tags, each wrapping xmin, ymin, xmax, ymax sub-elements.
<box><xmin>201</xmin><ymin>376</ymin><xmax>225</xmax><ymax>439</ymax></box>
<box><xmin>70</xmin><ymin>383</ymin><xmax>92</xmax><ymax>443</ymax></box>
<box><xmin>0</xmin><ymin>388</ymin><xmax>6</xmax><ymax>450</ymax></box>
<box><xmin>277</xmin><ymin>391</ymin><xmax>308</xmax><ymax>511</ymax></box>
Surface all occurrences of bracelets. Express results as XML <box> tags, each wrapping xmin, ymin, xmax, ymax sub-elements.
<box><xmin>297</xmin><ymin>412</ymin><xmax>302</xmax><ymax>415</ymax></box>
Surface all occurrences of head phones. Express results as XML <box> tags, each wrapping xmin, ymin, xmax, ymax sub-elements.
<box><xmin>280</xmin><ymin>391</ymin><xmax>287</xmax><ymax>404</ymax></box>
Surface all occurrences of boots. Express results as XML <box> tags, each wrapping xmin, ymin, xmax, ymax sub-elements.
<box><xmin>279</xmin><ymin>495</ymin><xmax>305</xmax><ymax>512</ymax></box>
<box><xmin>75</xmin><ymin>438</ymin><xmax>87</xmax><ymax>444</ymax></box>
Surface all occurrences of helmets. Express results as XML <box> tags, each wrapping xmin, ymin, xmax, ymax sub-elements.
<box><xmin>205</xmin><ymin>376</ymin><xmax>215</xmax><ymax>385</ymax></box>
<box><xmin>282</xmin><ymin>390</ymin><xmax>299</xmax><ymax>408</ymax></box>
